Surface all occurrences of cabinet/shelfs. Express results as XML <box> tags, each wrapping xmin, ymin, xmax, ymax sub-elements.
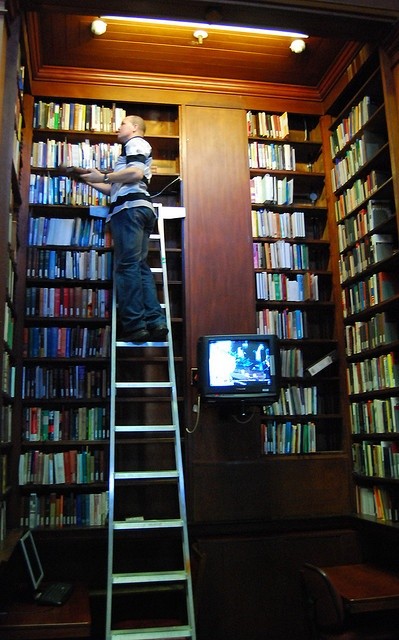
<box><xmin>15</xmin><ymin>96</ymin><xmax>190</xmax><ymax>532</ymax></box>
<box><xmin>243</xmin><ymin>107</ymin><xmax>348</xmax><ymax>461</ymax></box>
<box><xmin>327</xmin><ymin>32</ymin><xmax>398</xmax><ymax>527</ymax></box>
<box><xmin>1</xmin><ymin>0</ymin><xmax>32</xmax><ymax>542</ymax></box>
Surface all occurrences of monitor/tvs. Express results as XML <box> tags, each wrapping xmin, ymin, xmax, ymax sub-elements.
<box><xmin>198</xmin><ymin>331</ymin><xmax>287</xmax><ymax>408</ymax></box>
<box><xmin>20</xmin><ymin>529</ymin><xmax>49</xmax><ymax>592</ymax></box>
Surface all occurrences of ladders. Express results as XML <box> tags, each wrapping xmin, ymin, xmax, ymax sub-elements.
<box><xmin>106</xmin><ymin>201</ymin><xmax>199</xmax><ymax>640</ymax></box>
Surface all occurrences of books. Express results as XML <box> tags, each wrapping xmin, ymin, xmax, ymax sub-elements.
<box><xmin>25</xmin><ymin>287</ymin><xmax>109</xmax><ymax>319</ymax></box>
<box><xmin>280</xmin><ymin>348</ymin><xmax>303</xmax><ymax>377</ymax></box>
<box><xmin>0</xmin><ymin>501</ymin><xmax>7</xmax><ymax>541</ymax></box>
<box><xmin>29</xmin><ymin>174</ymin><xmax>112</xmax><ymax>207</ymax></box>
<box><xmin>27</xmin><ymin>248</ymin><xmax>111</xmax><ymax>280</ymax></box>
<box><xmin>22</xmin><ymin>325</ymin><xmax>111</xmax><ymax>358</ymax></box>
<box><xmin>246</xmin><ymin>111</ymin><xmax>289</xmax><ymax>139</ymax></box>
<box><xmin>256</xmin><ymin>272</ymin><xmax>319</xmax><ymax>302</ymax></box>
<box><xmin>356</xmin><ymin>485</ymin><xmax>398</xmax><ymax>523</ymax></box>
<box><xmin>28</xmin><ymin>217</ymin><xmax>111</xmax><ymax>249</ymax></box>
<box><xmin>350</xmin><ymin>396</ymin><xmax>399</xmax><ymax>434</ymax></box>
<box><xmin>251</xmin><ymin>209</ymin><xmax>305</xmax><ymax>239</ymax></box>
<box><xmin>32</xmin><ymin>101</ymin><xmax>126</xmax><ymax>133</ymax></box>
<box><xmin>346</xmin><ymin>357</ymin><xmax>399</xmax><ymax>396</ymax></box>
<box><xmin>20</xmin><ymin>491</ymin><xmax>109</xmax><ymax>528</ymax></box>
<box><xmin>3</xmin><ymin>42</ymin><xmax>22</xmax><ymax>350</ymax></box>
<box><xmin>351</xmin><ymin>441</ymin><xmax>399</xmax><ymax>480</ymax></box>
<box><xmin>22</xmin><ymin>406</ymin><xmax>110</xmax><ymax>442</ymax></box>
<box><xmin>30</xmin><ymin>139</ymin><xmax>122</xmax><ymax>171</ymax></box>
<box><xmin>0</xmin><ymin>454</ymin><xmax>7</xmax><ymax>495</ymax></box>
<box><xmin>1</xmin><ymin>405</ymin><xmax>12</xmax><ymax>443</ymax></box>
<box><xmin>261</xmin><ymin>421</ymin><xmax>316</xmax><ymax>455</ymax></box>
<box><xmin>22</xmin><ymin>366</ymin><xmax>106</xmax><ymax>399</ymax></box>
<box><xmin>304</xmin><ymin>349</ymin><xmax>339</xmax><ymax>378</ymax></box>
<box><xmin>253</xmin><ymin>240</ymin><xmax>309</xmax><ymax>271</ymax></box>
<box><xmin>2</xmin><ymin>351</ymin><xmax>15</xmax><ymax>398</ymax></box>
<box><xmin>263</xmin><ymin>384</ymin><xmax>317</xmax><ymax>416</ymax></box>
<box><xmin>256</xmin><ymin>309</ymin><xmax>304</xmax><ymax>340</ymax></box>
<box><xmin>248</xmin><ymin>142</ymin><xmax>296</xmax><ymax>172</ymax></box>
<box><xmin>330</xmin><ymin>96</ymin><xmax>399</xmax><ymax>357</ymax></box>
<box><xmin>19</xmin><ymin>446</ymin><xmax>104</xmax><ymax>485</ymax></box>
<box><xmin>249</xmin><ymin>174</ymin><xmax>294</xmax><ymax>205</ymax></box>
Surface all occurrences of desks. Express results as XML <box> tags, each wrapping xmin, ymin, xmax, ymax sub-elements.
<box><xmin>319</xmin><ymin>564</ymin><xmax>399</xmax><ymax>613</ymax></box>
<box><xmin>0</xmin><ymin>581</ymin><xmax>91</xmax><ymax>639</ymax></box>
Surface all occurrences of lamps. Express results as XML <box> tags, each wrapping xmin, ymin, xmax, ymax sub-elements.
<box><xmin>91</xmin><ymin>20</ymin><xmax>107</xmax><ymax>35</ymax></box>
<box><xmin>193</xmin><ymin>30</ymin><xmax>208</xmax><ymax>45</ymax></box>
<box><xmin>290</xmin><ymin>39</ymin><xmax>305</xmax><ymax>54</ymax></box>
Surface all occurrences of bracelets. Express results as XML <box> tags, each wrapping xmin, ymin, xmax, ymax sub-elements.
<box><xmin>103</xmin><ymin>173</ymin><xmax>109</xmax><ymax>184</ymax></box>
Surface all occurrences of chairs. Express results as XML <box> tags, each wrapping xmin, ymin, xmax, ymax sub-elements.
<box><xmin>302</xmin><ymin>561</ymin><xmax>359</xmax><ymax>639</ymax></box>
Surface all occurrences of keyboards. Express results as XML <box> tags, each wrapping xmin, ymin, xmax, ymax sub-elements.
<box><xmin>32</xmin><ymin>581</ymin><xmax>75</xmax><ymax>607</ymax></box>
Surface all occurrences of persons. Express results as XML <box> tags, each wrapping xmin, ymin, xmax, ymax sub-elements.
<box><xmin>80</xmin><ymin>115</ymin><xmax>169</xmax><ymax>342</ymax></box>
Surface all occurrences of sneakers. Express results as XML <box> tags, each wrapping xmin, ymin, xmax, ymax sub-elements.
<box><xmin>148</xmin><ymin>324</ymin><xmax>169</xmax><ymax>341</ymax></box>
<box><xmin>116</xmin><ymin>327</ymin><xmax>150</xmax><ymax>341</ymax></box>
